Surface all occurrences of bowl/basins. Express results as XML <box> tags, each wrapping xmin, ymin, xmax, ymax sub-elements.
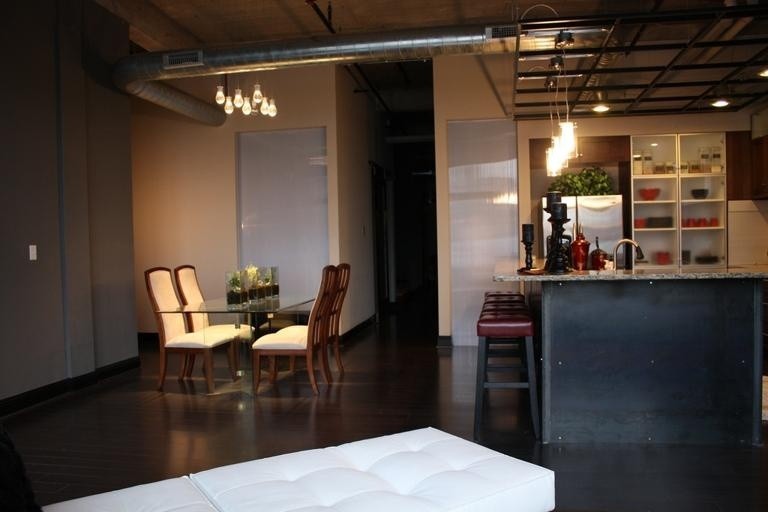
<box><xmin>634</xmin><ymin>219</ymin><xmax>649</xmax><ymax>228</ymax></box>
<box><xmin>691</xmin><ymin>189</ymin><xmax>710</xmax><ymax>199</ymax></box>
<box><xmin>638</xmin><ymin>187</ymin><xmax>661</xmax><ymax>200</ymax></box>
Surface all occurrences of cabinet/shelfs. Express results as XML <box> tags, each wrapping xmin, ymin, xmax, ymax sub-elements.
<box><xmin>678</xmin><ymin>131</ymin><xmax>729</xmax><ymax>266</ymax></box>
<box><xmin>630</xmin><ymin>133</ymin><xmax>680</xmax><ymax>270</ymax></box>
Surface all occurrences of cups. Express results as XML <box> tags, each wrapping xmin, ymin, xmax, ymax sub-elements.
<box><xmin>658</xmin><ymin>253</ymin><xmax>670</xmax><ymax>264</ymax></box>
<box><xmin>682</xmin><ymin>217</ymin><xmax>720</xmax><ymax>228</ymax></box>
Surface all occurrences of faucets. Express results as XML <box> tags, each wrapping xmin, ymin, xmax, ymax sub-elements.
<box><xmin>613</xmin><ymin>239</ymin><xmax>644</xmax><ymax>270</ymax></box>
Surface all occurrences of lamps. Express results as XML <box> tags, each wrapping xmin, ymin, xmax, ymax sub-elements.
<box><xmin>711</xmin><ymin>85</ymin><xmax>730</xmax><ymax>108</ymax></box>
<box><xmin>592</xmin><ymin>90</ymin><xmax>611</xmax><ymax>113</ymax></box>
<box><xmin>544</xmin><ymin>30</ymin><xmax>579</xmax><ymax>178</ymax></box>
<box><xmin>215</xmin><ymin>68</ymin><xmax>277</xmax><ymax>118</ymax></box>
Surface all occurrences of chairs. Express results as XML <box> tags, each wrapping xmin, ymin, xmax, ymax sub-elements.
<box><xmin>174</xmin><ymin>265</ymin><xmax>257</xmax><ymax>377</ymax></box>
<box><xmin>275</xmin><ymin>262</ymin><xmax>351</xmax><ymax>381</ymax></box>
<box><xmin>144</xmin><ymin>267</ymin><xmax>237</xmax><ymax>394</ymax></box>
<box><xmin>252</xmin><ymin>265</ymin><xmax>339</xmax><ymax>395</ymax></box>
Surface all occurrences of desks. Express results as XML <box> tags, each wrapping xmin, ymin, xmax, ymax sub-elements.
<box><xmin>155</xmin><ymin>296</ymin><xmax>315</xmax><ymax>397</ymax></box>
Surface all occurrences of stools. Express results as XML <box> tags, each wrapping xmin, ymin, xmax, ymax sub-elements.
<box><xmin>473</xmin><ymin>290</ymin><xmax>542</xmax><ymax>443</ymax></box>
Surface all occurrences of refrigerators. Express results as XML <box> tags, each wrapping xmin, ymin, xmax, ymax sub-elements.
<box><xmin>538</xmin><ymin>195</ymin><xmax>626</xmax><ymax>271</ymax></box>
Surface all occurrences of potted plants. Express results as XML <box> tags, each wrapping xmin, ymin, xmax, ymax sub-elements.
<box><xmin>225</xmin><ymin>264</ymin><xmax>279</xmax><ymax>305</ymax></box>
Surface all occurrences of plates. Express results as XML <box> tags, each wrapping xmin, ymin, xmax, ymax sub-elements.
<box><xmin>693</xmin><ymin>255</ymin><xmax>719</xmax><ymax>265</ymax></box>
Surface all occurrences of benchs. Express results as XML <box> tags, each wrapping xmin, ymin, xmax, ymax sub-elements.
<box><xmin>40</xmin><ymin>424</ymin><xmax>555</xmax><ymax>512</ymax></box>
